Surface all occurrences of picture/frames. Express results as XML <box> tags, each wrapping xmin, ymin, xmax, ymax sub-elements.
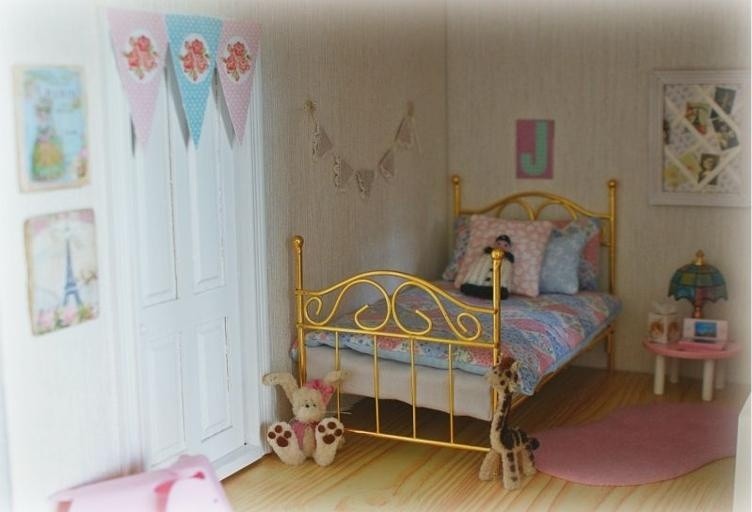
<box><xmin>10</xmin><ymin>63</ymin><xmax>91</xmax><ymax>193</ymax></box>
<box><xmin>645</xmin><ymin>68</ymin><xmax>751</xmax><ymax>208</ymax></box>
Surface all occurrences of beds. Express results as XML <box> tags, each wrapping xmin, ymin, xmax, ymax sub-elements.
<box><xmin>290</xmin><ymin>174</ymin><xmax>617</xmax><ymax>476</ymax></box>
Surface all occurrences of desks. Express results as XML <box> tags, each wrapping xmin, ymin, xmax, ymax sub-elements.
<box><xmin>644</xmin><ymin>332</ymin><xmax>739</xmax><ymax>402</ymax></box>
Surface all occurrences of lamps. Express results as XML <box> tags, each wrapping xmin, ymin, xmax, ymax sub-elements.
<box><xmin>669</xmin><ymin>253</ymin><xmax>729</xmax><ymax>328</ymax></box>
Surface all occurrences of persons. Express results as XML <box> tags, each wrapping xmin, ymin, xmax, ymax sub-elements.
<box><xmin>461</xmin><ymin>235</ymin><xmax>514</xmax><ymax>299</ymax></box>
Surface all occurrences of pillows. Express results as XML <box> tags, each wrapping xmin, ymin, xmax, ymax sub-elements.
<box><xmin>458</xmin><ymin>222</ymin><xmax>580</xmax><ymax>298</ymax></box>
<box><xmin>444</xmin><ymin>213</ymin><xmax>600</xmax><ymax>299</ymax></box>
<box><xmin>453</xmin><ymin>214</ymin><xmax>551</xmax><ymax>300</ymax></box>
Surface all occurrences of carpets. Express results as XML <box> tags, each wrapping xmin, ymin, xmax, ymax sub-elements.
<box><xmin>523</xmin><ymin>401</ymin><xmax>738</xmax><ymax>488</ymax></box>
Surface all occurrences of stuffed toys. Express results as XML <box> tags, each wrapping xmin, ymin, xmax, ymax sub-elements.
<box><xmin>478</xmin><ymin>357</ymin><xmax>540</xmax><ymax>492</ymax></box>
<box><xmin>262</xmin><ymin>369</ymin><xmax>353</xmax><ymax>467</ymax></box>
